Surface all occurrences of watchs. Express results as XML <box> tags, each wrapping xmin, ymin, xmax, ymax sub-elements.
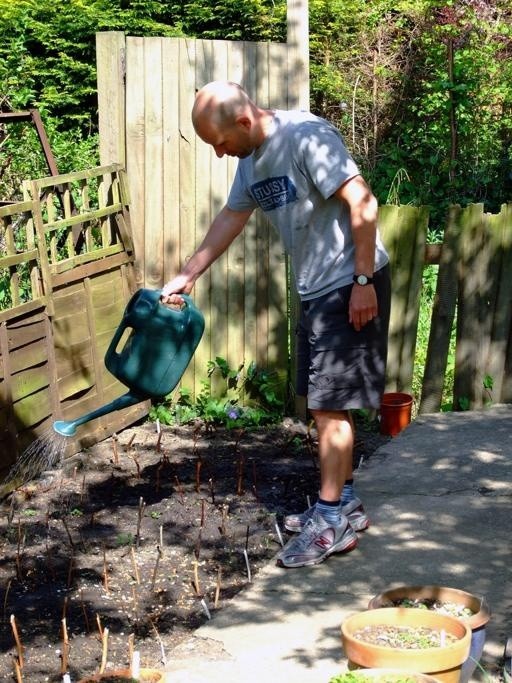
<box><xmin>354</xmin><ymin>274</ymin><xmax>374</xmax><ymax>286</ymax></box>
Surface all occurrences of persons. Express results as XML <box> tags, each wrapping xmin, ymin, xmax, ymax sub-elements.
<box><xmin>160</xmin><ymin>80</ymin><xmax>390</xmax><ymax>568</ymax></box>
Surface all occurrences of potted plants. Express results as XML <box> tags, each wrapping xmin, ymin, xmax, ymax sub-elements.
<box><xmin>329</xmin><ymin>586</ymin><xmax>492</xmax><ymax>683</ymax></box>
<box><xmin>77</xmin><ymin>668</ymin><xmax>162</xmax><ymax>683</ymax></box>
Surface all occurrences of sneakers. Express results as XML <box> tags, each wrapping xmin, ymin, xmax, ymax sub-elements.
<box><xmin>276</xmin><ymin>510</ymin><xmax>359</xmax><ymax>568</ymax></box>
<box><xmin>284</xmin><ymin>494</ymin><xmax>370</xmax><ymax>533</ymax></box>
